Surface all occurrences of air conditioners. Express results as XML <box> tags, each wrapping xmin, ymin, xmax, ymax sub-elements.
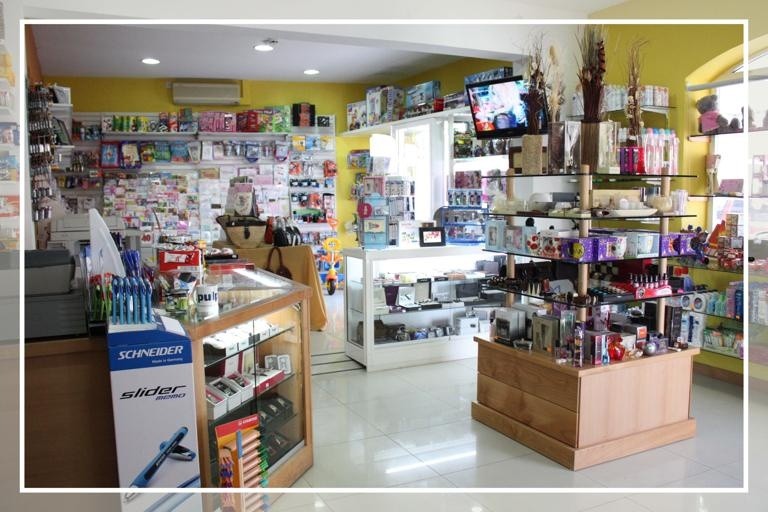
<box><xmin>170</xmin><ymin>79</ymin><xmax>245</xmax><ymax>108</ymax></box>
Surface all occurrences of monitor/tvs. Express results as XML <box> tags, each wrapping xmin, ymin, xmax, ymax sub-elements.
<box><xmin>466</xmin><ymin>72</ymin><xmax>549</xmax><ymax>140</ymax></box>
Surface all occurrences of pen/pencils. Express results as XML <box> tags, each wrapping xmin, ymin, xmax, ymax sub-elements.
<box><xmin>80</xmin><ymin>248</ymin><xmax>152</xmax><ymax>325</ymax></box>
<box><xmin>123</xmin><ymin>426</ymin><xmax>195</xmax><ymax>503</ymax></box>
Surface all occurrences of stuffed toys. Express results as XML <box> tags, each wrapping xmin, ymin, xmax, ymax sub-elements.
<box><xmin>696</xmin><ymin>95</ymin><xmax>739</xmax><ymax>135</ymax></box>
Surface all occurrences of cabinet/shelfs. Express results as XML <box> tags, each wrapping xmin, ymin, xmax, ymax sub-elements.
<box><xmin>338</xmin><ymin>243</ymin><xmax>502</xmax><ymax>374</ymax></box>
<box><xmin>466</xmin><ymin>169</ymin><xmax>720</xmax><ymax>477</ymax></box>
<box><xmin>183</xmin><ymin>264</ymin><xmax>316</xmax><ymax>511</ymax></box>
<box><xmin>100</xmin><ymin>130</ymin><xmax>288</xmax><ymax>170</ymax></box>
<box><xmin>681</xmin><ymin>65</ymin><xmax>768</xmax><ymax>393</ymax></box>
<box><xmin>49</xmin><ymin>100</ymin><xmax>74</xmax><ymax>161</ymax></box>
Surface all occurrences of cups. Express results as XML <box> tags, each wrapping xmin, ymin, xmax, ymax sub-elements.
<box><xmin>609</xmin><ymin>236</ymin><xmax>628</xmax><ymax>257</ymax></box>
<box><xmin>191</xmin><ymin>283</ymin><xmax>219</xmax><ymax>314</ymax></box>
<box><xmin>638</xmin><ymin>235</ymin><xmax>655</xmax><ymax>254</ymax></box>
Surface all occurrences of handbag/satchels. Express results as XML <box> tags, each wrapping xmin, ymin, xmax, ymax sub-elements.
<box><xmin>265</xmin><ymin>247</ymin><xmax>293</xmax><ymax>280</ymax></box>
